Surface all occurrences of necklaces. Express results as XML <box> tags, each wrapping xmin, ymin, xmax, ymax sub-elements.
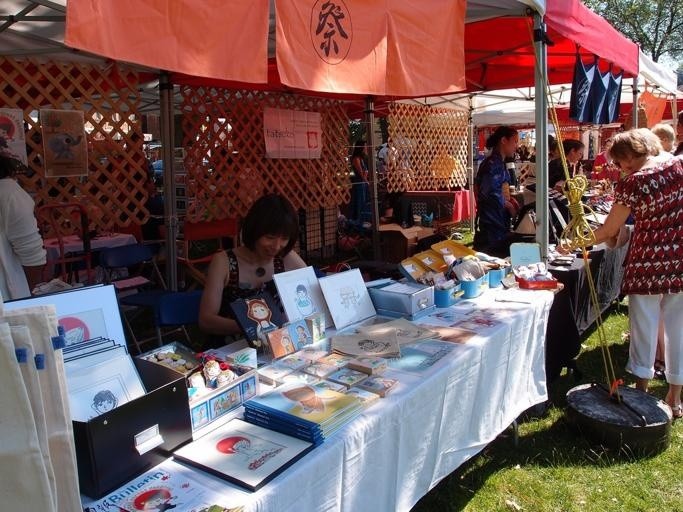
<box><xmin>236</xmin><ymin>250</ymin><xmax>268</xmax><ymax>276</ymax></box>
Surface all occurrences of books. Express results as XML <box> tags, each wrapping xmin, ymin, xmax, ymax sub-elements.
<box><xmin>82</xmin><ymin>467</ymin><xmax>244</xmax><ymax>512</ymax></box>
<box><xmin>173</xmin><ymin>417</ymin><xmax>315</xmax><ymax>490</ymax></box>
<box><xmin>358</xmin><ymin>317</ymin><xmax>441</xmax><ymax>345</ymax></box>
<box><xmin>271</xmin><ymin>265</ymin><xmax>335</xmax><ymax>329</ymax></box>
<box><xmin>242</xmin><ymin>383</ymin><xmax>363</xmax><ymax>446</ymax></box>
<box><xmin>331</xmin><ymin>328</ymin><xmax>401</xmax><ymax>361</ymax></box>
<box><xmin>318</xmin><ymin>267</ymin><xmax>376</xmax><ymax>328</ymax></box>
<box><xmin>228</xmin><ymin>290</ymin><xmax>279</xmax><ymax>352</ymax></box>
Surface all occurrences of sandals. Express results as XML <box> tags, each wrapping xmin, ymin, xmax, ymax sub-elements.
<box><xmin>654</xmin><ymin>359</ymin><xmax>683</xmax><ymax>417</ymax></box>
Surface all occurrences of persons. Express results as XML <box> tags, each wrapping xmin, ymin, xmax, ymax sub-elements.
<box><xmin>0</xmin><ymin>151</ymin><xmax>48</xmax><ymax>301</ymax></box>
<box><xmin>652</xmin><ymin>112</ymin><xmax>683</xmax><ymax>157</ymax></box>
<box><xmin>111</xmin><ymin>134</ymin><xmax>153</xmax><ymax>177</ymax></box>
<box><xmin>377</xmin><ymin>133</ymin><xmax>458</xmax><ymax>219</ymax></box>
<box><xmin>473</xmin><ymin>126</ymin><xmax>525</xmax><ymax>261</ymax></box>
<box><xmin>350</xmin><ymin>139</ymin><xmax>371</xmax><ymax>221</ymax></box>
<box><xmin>556</xmin><ymin>127</ymin><xmax>683</xmax><ymax>418</ymax></box>
<box><xmin>531</xmin><ymin>135</ymin><xmax>583</xmax><ymax>242</ymax></box>
<box><xmin>129</xmin><ymin>172</ymin><xmax>166</xmax><ymax>242</ymax></box>
<box><xmin>196</xmin><ymin>188</ymin><xmax>310</xmax><ymax>354</ymax></box>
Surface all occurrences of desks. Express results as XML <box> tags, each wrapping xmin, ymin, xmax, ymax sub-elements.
<box><xmin>55</xmin><ymin>163</ymin><xmax>683</xmax><ymax>512</ymax></box>
